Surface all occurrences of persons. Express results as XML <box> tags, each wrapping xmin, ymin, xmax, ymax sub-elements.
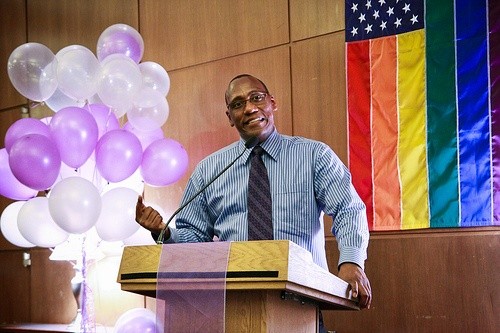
<box><xmin>136</xmin><ymin>74</ymin><xmax>372</xmax><ymax>333</ymax></box>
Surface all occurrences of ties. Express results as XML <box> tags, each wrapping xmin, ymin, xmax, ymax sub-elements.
<box><xmin>248</xmin><ymin>147</ymin><xmax>274</xmax><ymax>240</ymax></box>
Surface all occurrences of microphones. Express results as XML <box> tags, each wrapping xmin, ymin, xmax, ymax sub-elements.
<box><xmin>157</xmin><ymin>136</ymin><xmax>259</xmax><ymax>245</ymax></box>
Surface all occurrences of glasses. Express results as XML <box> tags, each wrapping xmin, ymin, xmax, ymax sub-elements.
<box><xmin>229</xmin><ymin>92</ymin><xmax>270</xmax><ymax>108</ymax></box>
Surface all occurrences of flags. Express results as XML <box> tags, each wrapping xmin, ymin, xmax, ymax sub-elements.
<box><xmin>344</xmin><ymin>0</ymin><xmax>500</xmax><ymax>233</ymax></box>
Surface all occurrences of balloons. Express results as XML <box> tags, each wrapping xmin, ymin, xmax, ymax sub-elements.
<box><xmin>0</xmin><ymin>23</ymin><xmax>189</xmax><ymax>293</ymax></box>
<box><xmin>116</xmin><ymin>307</ymin><xmax>162</xmax><ymax>333</ymax></box>
